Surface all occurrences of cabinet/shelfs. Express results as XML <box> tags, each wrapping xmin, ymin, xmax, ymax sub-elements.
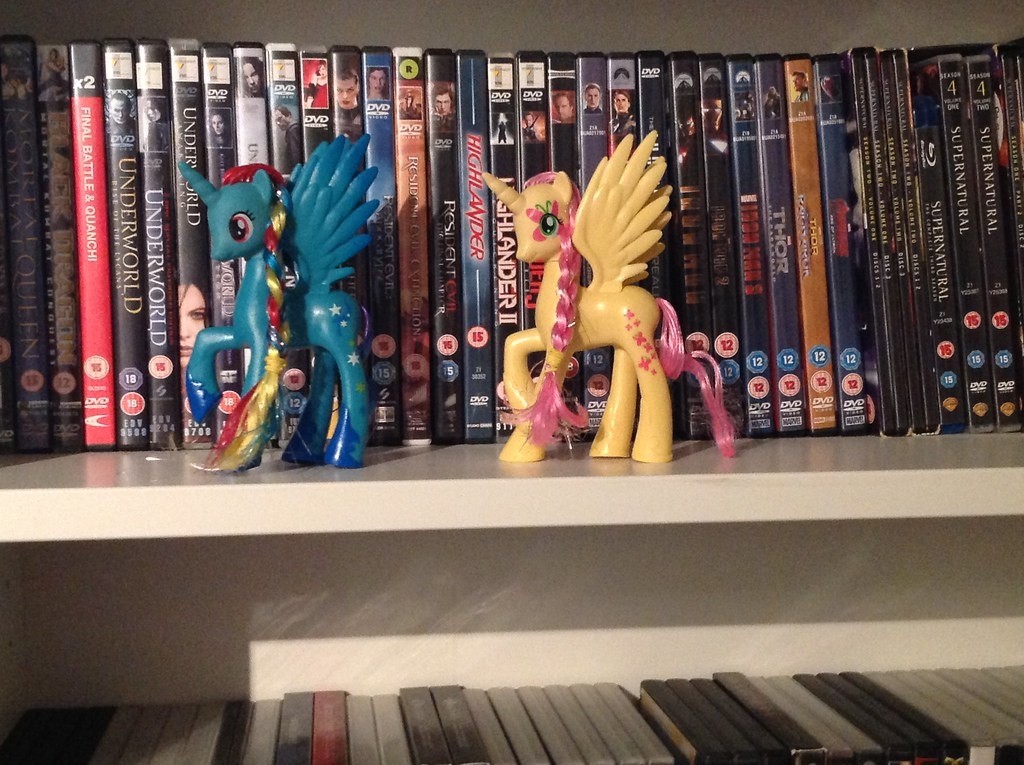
<box><xmin>0</xmin><ymin>1</ymin><xmax>1023</xmax><ymax>765</ymax></box>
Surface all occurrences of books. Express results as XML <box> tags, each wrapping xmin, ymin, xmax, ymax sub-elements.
<box><xmin>0</xmin><ymin>30</ymin><xmax>1024</xmax><ymax>454</ymax></box>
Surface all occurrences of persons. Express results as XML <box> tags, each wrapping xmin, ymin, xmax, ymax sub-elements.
<box><xmin>309</xmin><ymin>60</ymin><xmax>327</xmax><ymax>107</ymax></box>
<box><xmin>336</xmin><ymin>67</ymin><xmax>361</xmax><ymax>125</ymax></box>
<box><xmin>553</xmin><ymin>92</ymin><xmax>575</xmax><ymax>122</ymax></box>
<box><xmin>791</xmin><ymin>70</ymin><xmax>810</xmax><ymax>101</ymax></box>
<box><xmin>239</xmin><ymin>56</ymin><xmax>267</xmax><ymax>98</ymax></box>
<box><xmin>368</xmin><ymin>66</ymin><xmax>391</xmax><ymax>99</ymax></box>
<box><xmin>583</xmin><ymin>83</ymin><xmax>603</xmax><ymax>114</ymax></box>
<box><xmin>274</xmin><ymin>104</ymin><xmax>304</xmax><ymax>174</ymax></box>
<box><xmin>818</xmin><ymin>73</ymin><xmax>843</xmax><ymax>101</ymax></box>
<box><xmin>524</xmin><ymin>112</ymin><xmax>542</xmax><ymax>140</ymax></box>
<box><xmin>764</xmin><ymin>85</ymin><xmax>780</xmax><ymax>118</ymax></box>
<box><xmin>432</xmin><ymin>85</ymin><xmax>454</xmax><ymax>127</ymax></box>
<box><xmin>106</xmin><ymin>92</ymin><xmax>136</xmax><ymax>135</ymax></box>
<box><xmin>210</xmin><ymin>111</ymin><xmax>230</xmax><ymax>147</ymax></box>
<box><xmin>610</xmin><ymin>89</ymin><xmax>636</xmax><ymax>134</ymax></box>
